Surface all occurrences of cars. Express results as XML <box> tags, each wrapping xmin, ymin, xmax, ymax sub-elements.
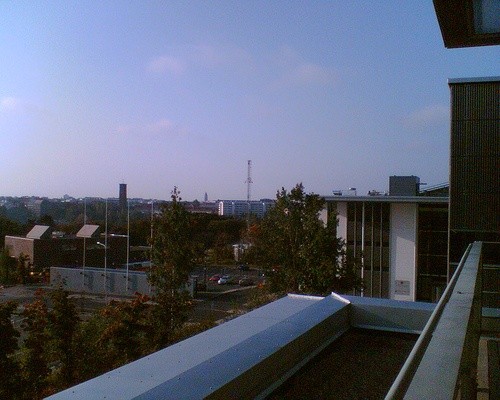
<box><xmin>217</xmin><ymin>275</ymin><xmax>234</xmax><ymax>285</ymax></box>
<box><xmin>208</xmin><ymin>274</ymin><xmax>220</xmax><ymax>282</ymax></box>
<box><xmin>239</xmin><ymin>278</ymin><xmax>254</xmax><ymax>286</ymax></box>
